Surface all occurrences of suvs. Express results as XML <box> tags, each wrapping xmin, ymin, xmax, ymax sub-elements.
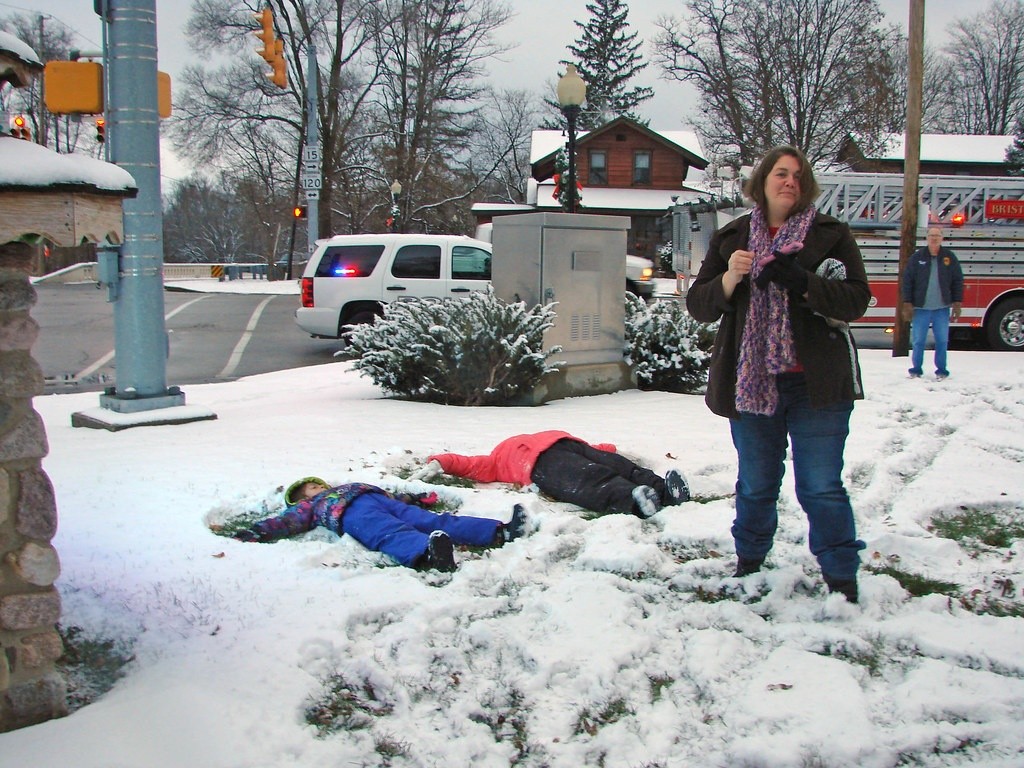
<box><xmin>274</xmin><ymin>252</ymin><xmax>305</xmax><ymax>272</ymax></box>
<box><xmin>293</xmin><ymin>234</ymin><xmax>493</xmax><ymax>348</ymax></box>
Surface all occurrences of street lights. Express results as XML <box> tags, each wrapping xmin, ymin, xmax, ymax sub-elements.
<box><xmin>558</xmin><ymin>64</ymin><xmax>586</xmax><ymax>214</ymax></box>
<box><xmin>391</xmin><ymin>179</ymin><xmax>403</xmax><ymax>234</ymax></box>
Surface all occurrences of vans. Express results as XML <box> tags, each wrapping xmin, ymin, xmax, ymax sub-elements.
<box><xmin>475</xmin><ymin>222</ymin><xmax>655</xmax><ymax>301</ymax></box>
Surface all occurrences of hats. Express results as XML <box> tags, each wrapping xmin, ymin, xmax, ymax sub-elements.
<box><xmin>812</xmin><ymin>257</ymin><xmax>861</xmax><ymax>394</ymax></box>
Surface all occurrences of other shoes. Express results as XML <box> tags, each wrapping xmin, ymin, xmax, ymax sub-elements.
<box><xmin>495</xmin><ymin>503</ymin><xmax>530</xmax><ymax>548</ymax></box>
<box><xmin>934</xmin><ymin>369</ymin><xmax>949</xmax><ymax>377</ymax></box>
<box><xmin>422</xmin><ymin>530</ymin><xmax>459</xmax><ymax>573</ymax></box>
<box><xmin>827</xmin><ymin>580</ymin><xmax>858</xmax><ymax>603</ymax></box>
<box><xmin>664</xmin><ymin>470</ymin><xmax>691</xmax><ymax>506</ymax></box>
<box><xmin>731</xmin><ymin>552</ymin><xmax>766</xmax><ymax>578</ymax></box>
<box><xmin>908</xmin><ymin>367</ymin><xmax>923</xmax><ymax>375</ymax></box>
<box><xmin>632</xmin><ymin>485</ymin><xmax>662</xmax><ymax>520</ymax></box>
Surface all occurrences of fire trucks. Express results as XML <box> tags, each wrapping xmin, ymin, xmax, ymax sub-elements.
<box><xmin>668</xmin><ymin>165</ymin><xmax>1024</xmax><ymax>351</ymax></box>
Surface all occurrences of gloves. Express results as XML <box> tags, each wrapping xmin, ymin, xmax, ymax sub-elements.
<box><xmin>232</xmin><ymin>524</ymin><xmax>268</xmax><ymax>543</ymax></box>
<box><xmin>900</xmin><ymin>302</ymin><xmax>914</xmax><ymax>322</ymax></box>
<box><xmin>405</xmin><ymin>458</ymin><xmax>448</xmax><ymax>482</ymax></box>
<box><xmin>615</xmin><ymin>448</ymin><xmax>642</xmax><ymax>465</ymax></box>
<box><xmin>754</xmin><ymin>249</ymin><xmax>808</xmax><ymax>297</ymax></box>
<box><xmin>951</xmin><ymin>302</ymin><xmax>961</xmax><ymax>318</ymax></box>
<box><xmin>415</xmin><ymin>492</ymin><xmax>438</xmax><ymax>505</ymax></box>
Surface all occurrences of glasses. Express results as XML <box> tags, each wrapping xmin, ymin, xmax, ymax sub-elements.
<box><xmin>928</xmin><ymin>233</ymin><xmax>942</xmax><ymax>239</ymax></box>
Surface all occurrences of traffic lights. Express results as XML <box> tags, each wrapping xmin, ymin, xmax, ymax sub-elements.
<box><xmin>266</xmin><ymin>39</ymin><xmax>287</xmax><ymax>89</ymax></box>
<box><xmin>21</xmin><ymin>127</ymin><xmax>31</xmax><ymax>142</ymax></box>
<box><xmin>97</xmin><ymin>118</ymin><xmax>105</xmax><ymax>143</ymax></box>
<box><xmin>16</xmin><ymin>117</ymin><xmax>25</xmax><ymax>127</ymax></box>
<box><xmin>294</xmin><ymin>207</ymin><xmax>306</xmax><ymax>218</ymax></box>
<box><xmin>9</xmin><ymin>128</ymin><xmax>20</xmax><ymax>138</ymax></box>
<box><xmin>255</xmin><ymin>9</ymin><xmax>275</xmax><ymax>63</ymax></box>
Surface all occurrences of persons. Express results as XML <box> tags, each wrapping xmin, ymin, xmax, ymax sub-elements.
<box><xmin>406</xmin><ymin>430</ymin><xmax>690</xmax><ymax>519</ymax></box>
<box><xmin>684</xmin><ymin>146</ymin><xmax>873</xmax><ymax>602</ymax></box>
<box><xmin>901</xmin><ymin>228</ymin><xmax>964</xmax><ymax>380</ymax></box>
<box><xmin>231</xmin><ymin>477</ymin><xmax>529</xmax><ymax>573</ymax></box>
